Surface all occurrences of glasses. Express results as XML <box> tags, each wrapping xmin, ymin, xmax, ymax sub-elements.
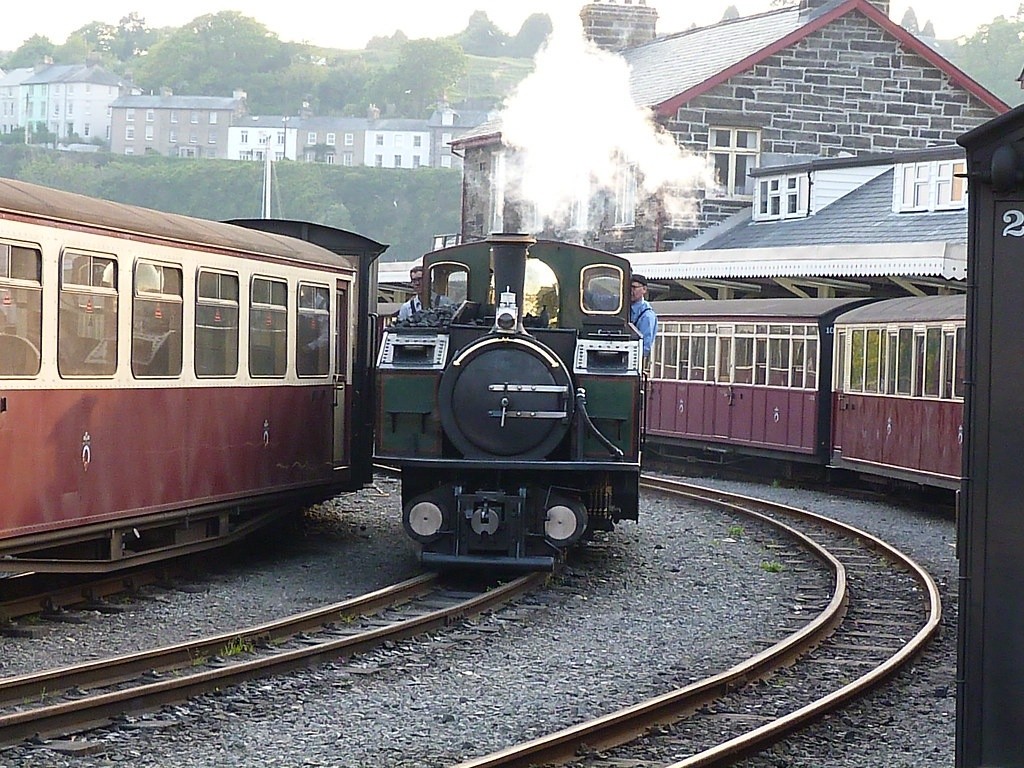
<box><xmin>632</xmin><ymin>286</ymin><xmax>643</xmax><ymax>289</ymax></box>
<box><xmin>411</xmin><ymin>277</ymin><xmax>423</xmax><ymax>283</ymax></box>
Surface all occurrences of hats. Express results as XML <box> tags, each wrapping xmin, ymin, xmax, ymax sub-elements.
<box><xmin>632</xmin><ymin>274</ymin><xmax>647</xmax><ymax>286</ymax></box>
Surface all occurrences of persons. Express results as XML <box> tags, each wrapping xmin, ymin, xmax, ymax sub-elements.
<box><xmin>584</xmin><ymin>274</ymin><xmax>658</xmax><ymax>358</ymax></box>
<box><xmin>297</xmin><ymin>284</ymin><xmax>329</xmax><ymax>375</ymax></box>
<box><xmin>396</xmin><ymin>266</ymin><xmax>457</xmax><ymax>323</ymax></box>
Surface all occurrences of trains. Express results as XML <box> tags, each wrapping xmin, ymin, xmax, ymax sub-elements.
<box><xmin>645</xmin><ymin>295</ymin><xmax>966</xmax><ymax>496</ymax></box>
<box><xmin>2</xmin><ymin>181</ymin><xmax>376</xmax><ymax>579</ymax></box>
<box><xmin>373</xmin><ymin>234</ymin><xmax>646</xmax><ymax>572</ymax></box>
